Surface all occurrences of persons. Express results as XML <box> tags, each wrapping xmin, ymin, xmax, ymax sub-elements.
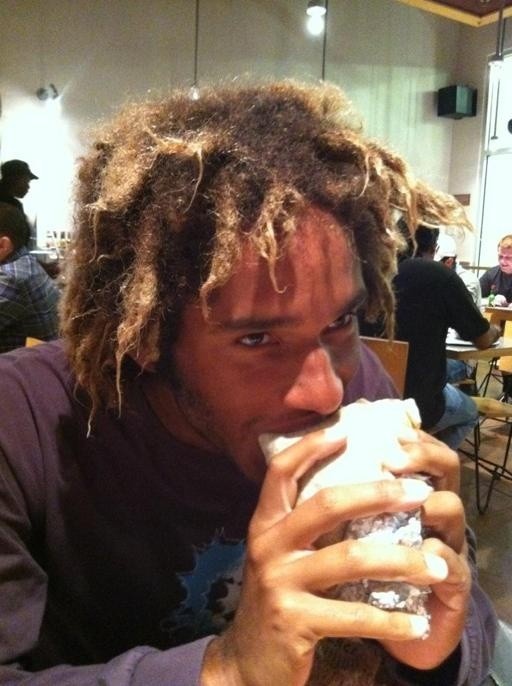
<box><xmin>0</xmin><ymin>159</ymin><xmax>64</xmax><ymax>351</ymax></box>
<box><xmin>393</xmin><ymin>213</ymin><xmax>512</xmax><ymax>451</ymax></box>
<box><xmin>0</xmin><ymin>72</ymin><xmax>497</xmax><ymax>686</ymax></box>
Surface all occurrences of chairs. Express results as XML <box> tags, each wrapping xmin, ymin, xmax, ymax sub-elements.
<box><xmin>357</xmin><ymin>332</ymin><xmax>511</xmax><ymax>514</ymax></box>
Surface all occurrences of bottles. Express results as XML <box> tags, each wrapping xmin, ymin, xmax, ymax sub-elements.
<box><xmin>488</xmin><ymin>285</ymin><xmax>495</xmax><ymax>307</ymax></box>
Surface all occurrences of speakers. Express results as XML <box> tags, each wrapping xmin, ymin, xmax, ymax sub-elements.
<box><xmin>438</xmin><ymin>84</ymin><xmax>476</xmax><ymax>119</ymax></box>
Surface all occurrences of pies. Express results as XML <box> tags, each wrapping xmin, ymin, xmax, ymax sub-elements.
<box><xmin>258</xmin><ymin>396</ymin><xmax>423</xmax><ymax>510</ymax></box>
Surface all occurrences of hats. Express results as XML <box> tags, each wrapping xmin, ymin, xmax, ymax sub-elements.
<box><xmin>0</xmin><ymin>159</ymin><xmax>39</xmax><ymax>181</ymax></box>
